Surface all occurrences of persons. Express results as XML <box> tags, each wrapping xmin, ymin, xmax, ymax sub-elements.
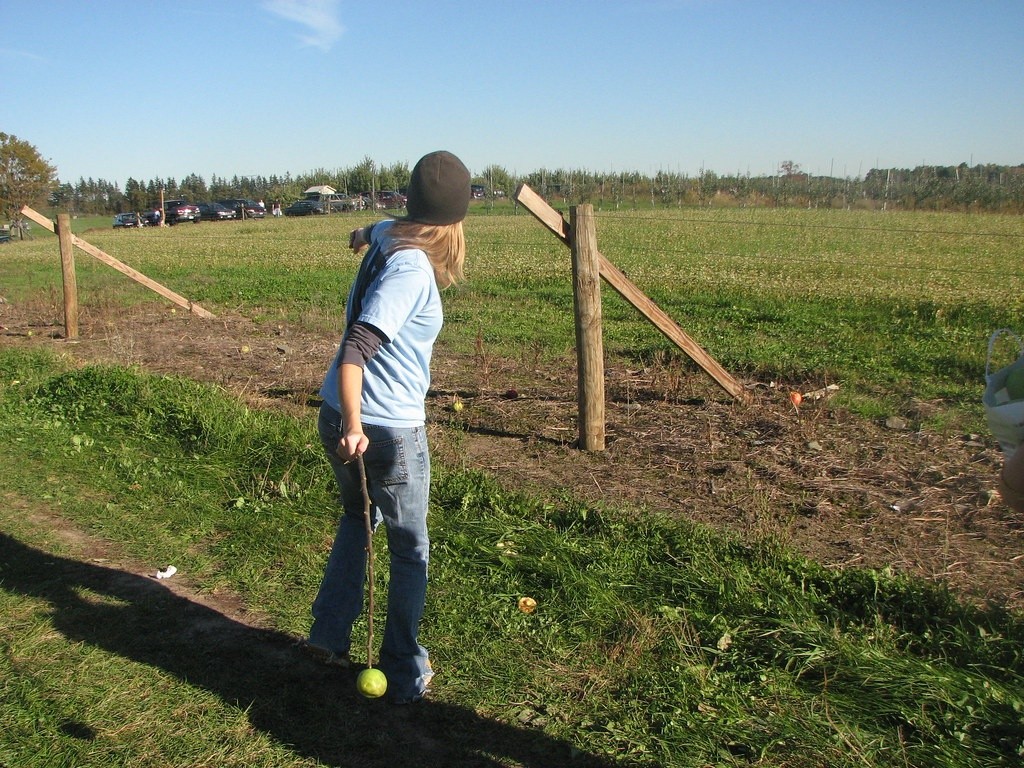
<box><xmin>258</xmin><ymin>196</ymin><xmax>281</xmax><ymax>217</ymax></box>
<box><xmin>308</xmin><ymin>150</ymin><xmax>471</xmax><ymax>706</ymax></box>
<box><xmin>998</xmin><ymin>444</ymin><xmax>1024</xmax><ymax>514</ymax></box>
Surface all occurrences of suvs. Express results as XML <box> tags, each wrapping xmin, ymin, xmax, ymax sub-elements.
<box><xmin>163</xmin><ymin>199</ymin><xmax>202</xmax><ymax>225</ymax></box>
<box><xmin>284</xmin><ymin>192</ymin><xmax>342</xmax><ymax>216</ymax></box>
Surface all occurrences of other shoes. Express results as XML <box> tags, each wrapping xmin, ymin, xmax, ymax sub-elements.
<box><xmin>313</xmin><ymin>645</ymin><xmax>349</xmax><ymax>665</ymax></box>
<box><xmin>391</xmin><ymin>659</ymin><xmax>432</xmax><ymax>704</ymax></box>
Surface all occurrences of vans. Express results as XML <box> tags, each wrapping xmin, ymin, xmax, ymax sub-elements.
<box><xmin>220</xmin><ymin>198</ymin><xmax>268</xmax><ymax>218</ymax></box>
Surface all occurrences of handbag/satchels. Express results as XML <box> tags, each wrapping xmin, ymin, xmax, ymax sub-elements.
<box><xmin>982</xmin><ymin>329</ymin><xmax>1024</xmax><ymax>460</ymax></box>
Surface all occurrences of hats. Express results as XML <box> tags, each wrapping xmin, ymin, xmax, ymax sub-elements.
<box><xmin>406</xmin><ymin>151</ymin><xmax>471</xmax><ymax>225</ymax></box>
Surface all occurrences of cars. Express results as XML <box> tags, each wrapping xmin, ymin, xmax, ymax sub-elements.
<box><xmin>330</xmin><ymin>191</ymin><xmax>408</xmax><ymax>213</ymax></box>
<box><xmin>112</xmin><ymin>212</ymin><xmax>141</xmax><ymax>229</ymax></box>
<box><xmin>197</xmin><ymin>202</ymin><xmax>237</xmax><ymax>221</ymax></box>
<box><xmin>471</xmin><ymin>186</ymin><xmax>486</xmax><ymax>200</ymax></box>
<box><xmin>140</xmin><ymin>210</ymin><xmax>163</xmax><ymax>226</ymax></box>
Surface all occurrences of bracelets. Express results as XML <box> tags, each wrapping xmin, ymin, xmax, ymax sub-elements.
<box><xmin>999</xmin><ymin>469</ymin><xmax>1019</xmax><ymax>495</ymax></box>
